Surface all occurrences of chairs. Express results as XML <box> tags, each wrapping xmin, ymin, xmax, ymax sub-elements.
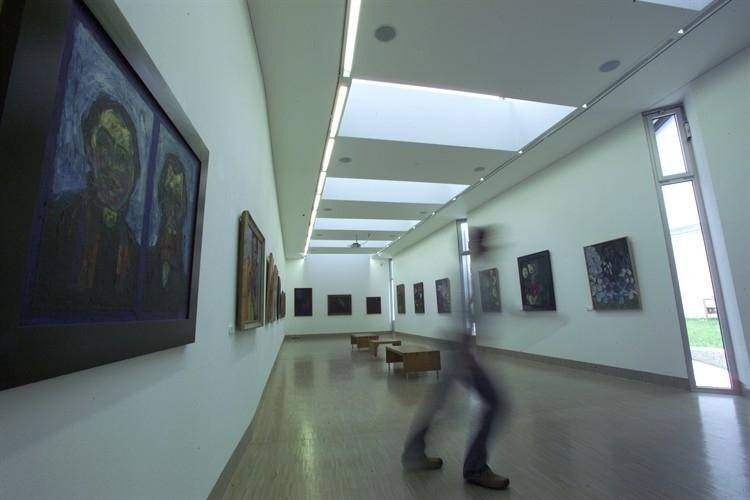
<box><xmin>703</xmin><ymin>298</ymin><xmax>718</xmax><ymax>319</ymax></box>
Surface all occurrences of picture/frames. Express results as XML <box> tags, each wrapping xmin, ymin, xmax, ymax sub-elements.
<box><xmin>236</xmin><ymin>209</ymin><xmax>286</xmax><ymax>332</ymax></box>
<box><xmin>0</xmin><ymin>0</ymin><xmax>209</xmax><ymax>391</ymax></box>
<box><xmin>396</xmin><ymin>249</ymin><xmax>557</xmax><ymax>314</ymax></box>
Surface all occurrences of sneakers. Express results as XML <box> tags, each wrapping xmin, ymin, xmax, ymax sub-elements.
<box><xmin>404</xmin><ymin>455</ymin><xmax>442</xmax><ymax>471</ymax></box>
<box><xmin>469</xmin><ymin>467</ymin><xmax>511</xmax><ymax>489</ymax></box>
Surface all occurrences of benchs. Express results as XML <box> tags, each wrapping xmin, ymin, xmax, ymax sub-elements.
<box><xmin>349</xmin><ymin>333</ymin><xmax>441</xmax><ymax>382</ymax></box>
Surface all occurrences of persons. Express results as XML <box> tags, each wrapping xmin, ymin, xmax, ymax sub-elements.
<box><xmin>30</xmin><ymin>90</ymin><xmax>143</xmax><ymax>311</ymax></box>
<box><xmin>144</xmin><ymin>152</ymin><xmax>190</xmax><ymax>314</ymax></box>
<box><xmin>521</xmin><ymin>265</ymin><xmax>535</xmax><ymax>303</ymax></box>
<box><xmin>526</xmin><ymin>262</ymin><xmax>546</xmax><ymax>307</ymax></box>
<box><xmin>402</xmin><ymin>226</ymin><xmax>509</xmax><ymax>490</ymax></box>
